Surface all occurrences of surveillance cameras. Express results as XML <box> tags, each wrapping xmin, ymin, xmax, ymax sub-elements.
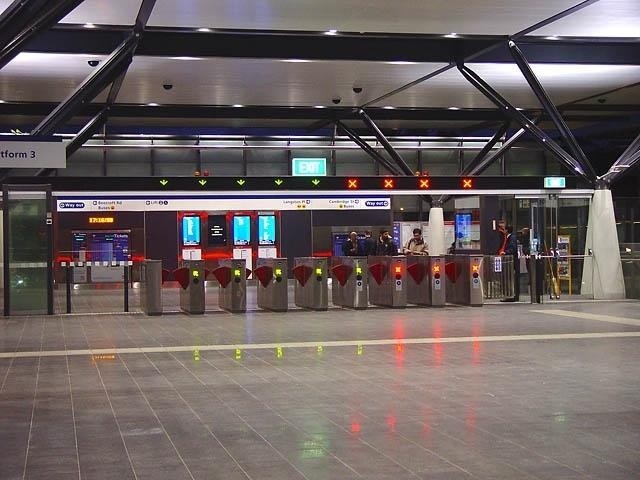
<box><xmin>163</xmin><ymin>85</ymin><xmax>172</xmax><ymax>90</ymax></box>
<box><xmin>87</xmin><ymin>61</ymin><xmax>99</xmax><ymax>67</ymax></box>
<box><xmin>332</xmin><ymin>99</ymin><xmax>340</xmax><ymax>104</ymax></box>
<box><xmin>353</xmin><ymin>88</ymin><xmax>362</xmax><ymax>94</ymax></box>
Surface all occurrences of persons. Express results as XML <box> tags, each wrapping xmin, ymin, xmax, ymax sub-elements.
<box><xmin>402</xmin><ymin>228</ymin><xmax>430</xmax><ymax>256</ymax></box>
<box><xmin>516</xmin><ymin>227</ymin><xmax>531</xmax><ymax>285</ymax></box>
<box><xmin>342</xmin><ymin>231</ymin><xmax>364</xmax><ymax>257</ymax></box>
<box><xmin>500</xmin><ymin>223</ymin><xmax>521</xmax><ymax>303</ymax></box>
<box><xmin>361</xmin><ymin>229</ymin><xmax>377</xmax><ymax>256</ymax></box>
<box><xmin>375</xmin><ymin>229</ymin><xmax>398</xmax><ymax>256</ymax></box>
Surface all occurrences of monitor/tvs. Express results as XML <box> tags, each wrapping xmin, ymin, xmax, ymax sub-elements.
<box><xmin>72</xmin><ymin>230</ymin><xmax>132</xmax><ymax>261</ymax></box>
<box><xmin>332</xmin><ymin>231</ymin><xmax>372</xmax><ymax>256</ymax></box>
